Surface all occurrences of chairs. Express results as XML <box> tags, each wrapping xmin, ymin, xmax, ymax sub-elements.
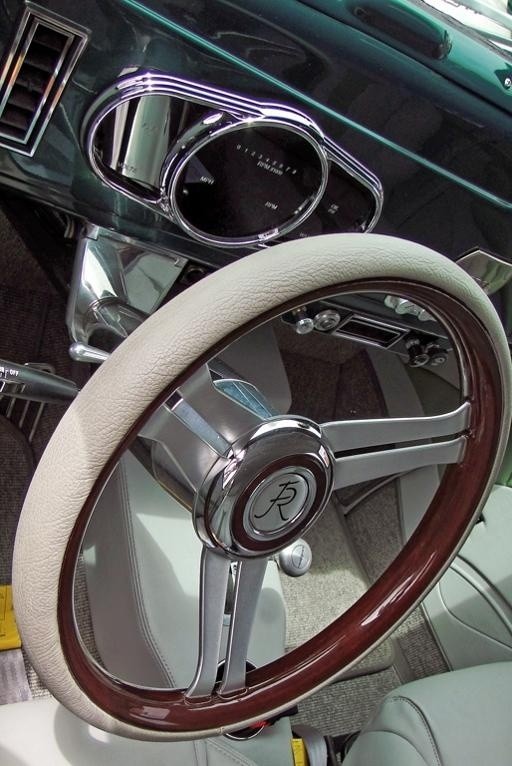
<box><xmin>338</xmin><ymin>651</ymin><xmax>510</xmax><ymax>766</ymax></box>
